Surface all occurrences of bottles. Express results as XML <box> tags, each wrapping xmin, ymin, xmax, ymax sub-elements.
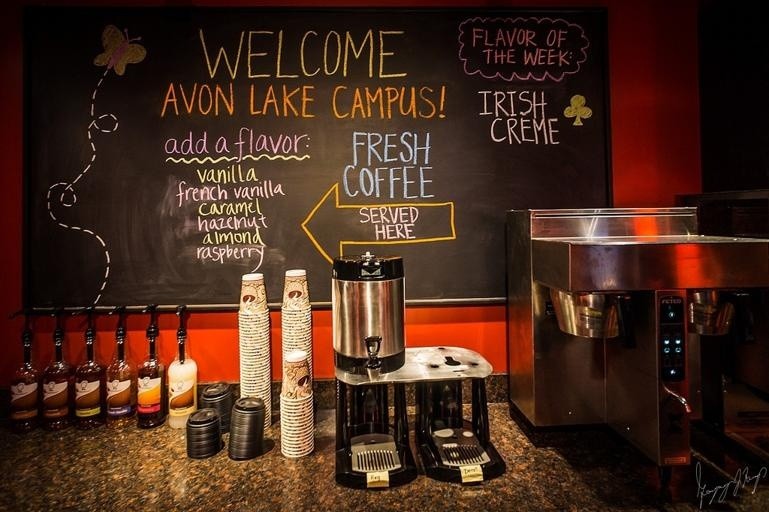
<box><xmin>71</xmin><ymin>325</ymin><xmax>105</xmax><ymax>430</ymax></box>
<box><xmin>168</xmin><ymin>326</ymin><xmax>198</xmax><ymax>427</ymax></box>
<box><xmin>137</xmin><ymin>327</ymin><xmax>165</xmax><ymax>428</ymax></box>
<box><xmin>42</xmin><ymin>327</ymin><xmax>72</xmax><ymax>430</ymax></box>
<box><xmin>8</xmin><ymin>328</ymin><xmax>40</xmax><ymax>432</ymax></box>
<box><xmin>104</xmin><ymin>323</ymin><xmax>130</xmax><ymax>418</ymax></box>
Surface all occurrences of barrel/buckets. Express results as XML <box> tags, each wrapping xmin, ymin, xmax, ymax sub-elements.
<box><xmin>330</xmin><ymin>252</ymin><xmax>407</xmax><ymax>375</ymax></box>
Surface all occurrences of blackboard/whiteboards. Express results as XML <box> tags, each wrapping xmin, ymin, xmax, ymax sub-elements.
<box><xmin>20</xmin><ymin>6</ymin><xmax>613</xmax><ymax>315</ymax></box>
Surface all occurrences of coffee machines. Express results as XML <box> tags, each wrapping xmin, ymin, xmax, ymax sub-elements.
<box><xmin>331</xmin><ymin>251</ymin><xmax>507</xmax><ymax>488</ymax></box>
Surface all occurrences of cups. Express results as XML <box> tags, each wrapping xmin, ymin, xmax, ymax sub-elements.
<box><xmin>228</xmin><ymin>397</ymin><xmax>267</xmax><ymax>461</ymax></box>
<box><xmin>238</xmin><ymin>273</ymin><xmax>273</xmax><ymax>431</ymax></box>
<box><xmin>186</xmin><ymin>408</ymin><xmax>222</xmax><ymax>460</ymax></box>
<box><xmin>280</xmin><ymin>352</ymin><xmax>315</xmax><ymax>458</ymax></box>
<box><xmin>282</xmin><ymin>268</ymin><xmax>313</xmax><ymax>379</ymax></box>
<box><xmin>199</xmin><ymin>381</ymin><xmax>232</xmax><ymax>433</ymax></box>
